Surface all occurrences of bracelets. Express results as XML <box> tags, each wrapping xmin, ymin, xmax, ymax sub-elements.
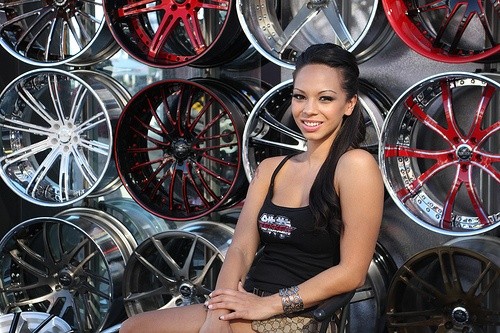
<box><xmin>278</xmin><ymin>285</ymin><xmax>305</xmax><ymax>316</ymax></box>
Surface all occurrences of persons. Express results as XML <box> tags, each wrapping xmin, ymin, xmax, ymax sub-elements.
<box><xmin>117</xmin><ymin>43</ymin><xmax>385</xmax><ymax>333</ymax></box>
